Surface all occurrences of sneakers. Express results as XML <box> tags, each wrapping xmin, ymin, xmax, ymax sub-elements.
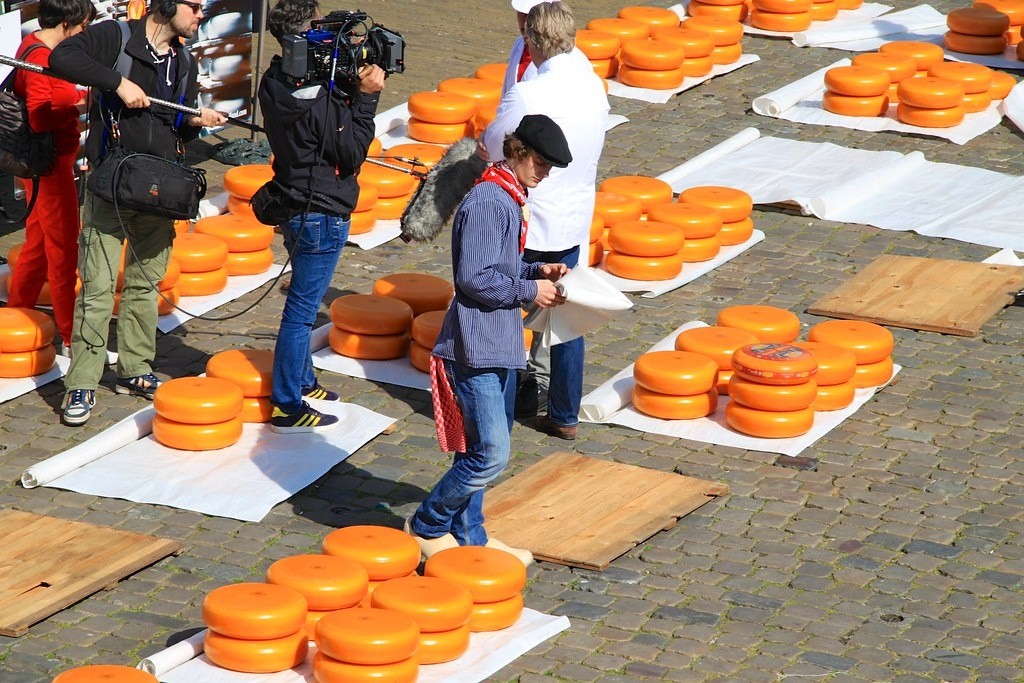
<box><xmin>299</xmin><ymin>376</ymin><xmax>339</xmax><ymax>402</ymax></box>
<box><xmin>270</xmin><ymin>400</ymin><xmax>339</xmax><ymax>434</ymax></box>
<box><xmin>63</xmin><ymin>388</ymin><xmax>95</xmax><ymax>425</ymax></box>
<box><xmin>114</xmin><ymin>372</ymin><xmax>164</xmax><ymax>399</ymax></box>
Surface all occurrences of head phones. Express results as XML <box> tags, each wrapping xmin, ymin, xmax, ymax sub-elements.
<box><xmin>159</xmin><ymin>0</ymin><xmax>177</xmax><ymax>17</ymax></box>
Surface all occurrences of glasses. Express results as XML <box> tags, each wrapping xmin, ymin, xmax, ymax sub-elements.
<box><xmin>79</xmin><ymin>21</ymin><xmax>90</xmax><ymax>30</ymax></box>
<box><xmin>171</xmin><ymin>0</ymin><xmax>202</xmax><ymax>13</ymax></box>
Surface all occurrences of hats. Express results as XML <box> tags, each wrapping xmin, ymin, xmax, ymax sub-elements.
<box><xmin>515</xmin><ymin>115</ymin><xmax>574</xmax><ymax>168</ymax></box>
<box><xmin>511</xmin><ymin>0</ymin><xmax>560</xmax><ymax>13</ymax></box>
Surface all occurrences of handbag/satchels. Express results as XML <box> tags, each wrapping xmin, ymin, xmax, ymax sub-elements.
<box><xmin>87</xmin><ymin>154</ymin><xmax>199</xmax><ymax>219</ymax></box>
<box><xmin>0</xmin><ymin>43</ymin><xmax>56</xmax><ymax>225</ymax></box>
<box><xmin>249</xmin><ymin>181</ymin><xmax>306</xmax><ymax>226</ymax></box>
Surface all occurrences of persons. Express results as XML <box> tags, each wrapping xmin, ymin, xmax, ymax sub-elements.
<box><xmin>475</xmin><ymin>0</ymin><xmax>611</xmax><ymax>440</ymax></box>
<box><xmin>0</xmin><ymin>0</ymin><xmax>118</xmax><ymax>365</ymax></box>
<box><xmin>258</xmin><ymin>0</ymin><xmax>386</xmax><ymax>433</ymax></box>
<box><xmin>48</xmin><ymin>0</ymin><xmax>227</xmax><ymax>427</ymax></box>
<box><xmin>405</xmin><ymin>115</ymin><xmax>574</xmax><ymax>568</ymax></box>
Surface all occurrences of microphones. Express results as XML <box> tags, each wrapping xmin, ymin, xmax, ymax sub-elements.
<box><xmin>399</xmin><ymin>138</ymin><xmax>488</xmax><ymax>244</ymax></box>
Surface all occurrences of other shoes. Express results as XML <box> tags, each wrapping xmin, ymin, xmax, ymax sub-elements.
<box><xmin>60</xmin><ymin>342</ymin><xmax>119</xmax><ymax>367</ymax></box>
<box><xmin>542</xmin><ymin>414</ymin><xmax>576</xmax><ymax>440</ymax></box>
<box><xmin>404</xmin><ymin>517</ymin><xmax>460</xmax><ymax>560</ymax></box>
<box><xmin>513</xmin><ymin>376</ymin><xmax>548</xmax><ymax>420</ymax></box>
<box><xmin>484</xmin><ymin>538</ymin><xmax>533</xmax><ymax>569</ymax></box>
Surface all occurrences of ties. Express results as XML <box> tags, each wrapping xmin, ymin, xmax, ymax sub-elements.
<box><xmin>479</xmin><ymin>163</ymin><xmax>529</xmax><ymax>254</ymax></box>
